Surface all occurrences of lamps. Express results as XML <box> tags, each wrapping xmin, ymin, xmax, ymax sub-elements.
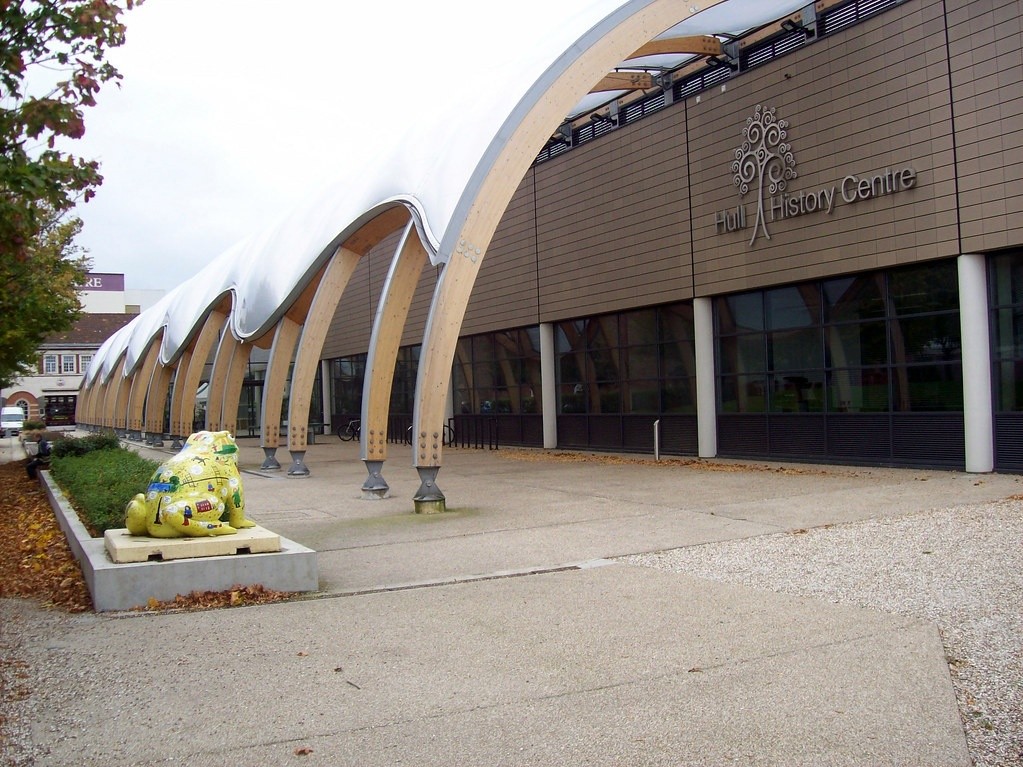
<box><xmin>549</xmin><ymin>137</ymin><xmax>572</xmax><ymax>147</ymax></box>
<box><xmin>591</xmin><ymin>113</ymin><xmax>618</xmax><ymax>126</ymax></box>
<box><xmin>707</xmin><ymin>57</ymin><xmax>739</xmax><ymax>71</ymax></box>
<box><xmin>781</xmin><ymin>19</ymin><xmax>816</xmax><ymax>38</ymax></box>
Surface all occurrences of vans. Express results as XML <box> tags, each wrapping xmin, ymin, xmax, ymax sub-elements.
<box><xmin>0</xmin><ymin>407</ymin><xmax>25</xmax><ymax>436</ymax></box>
